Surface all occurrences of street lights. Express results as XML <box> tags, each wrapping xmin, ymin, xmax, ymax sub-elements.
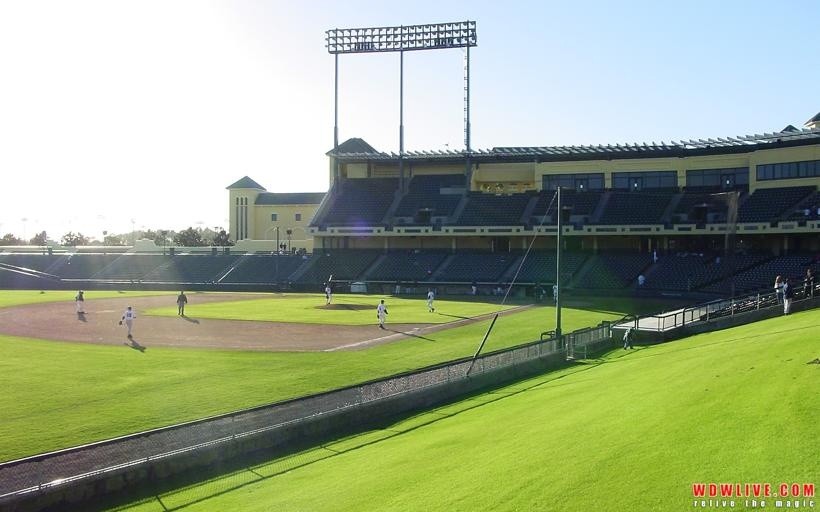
<box><xmin>325</xmin><ymin>20</ymin><xmax>478</xmax><ymax>198</ymax></box>
<box><xmin>102</xmin><ymin>232</ymin><xmax>107</xmax><ymax>255</ymax></box>
<box><xmin>219</xmin><ymin>231</ymin><xmax>227</xmax><ymax>254</ymax></box>
<box><xmin>286</xmin><ymin>229</ymin><xmax>292</xmax><ymax>253</ymax></box>
<box><xmin>161</xmin><ymin>230</ymin><xmax>167</xmax><ymax>256</ymax></box>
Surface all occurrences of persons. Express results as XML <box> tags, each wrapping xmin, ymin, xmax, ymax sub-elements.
<box><xmin>271</xmin><ymin>247</ymin><xmax>307</xmax><ymax>257</ymax></box>
<box><xmin>325</xmin><ymin>285</ymin><xmax>331</xmax><ymax>305</ymax></box>
<box><xmin>783</xmin><ymin>277</ymin><xmax>794</xmax><ymax>315</ymax></box>
<box><xmin>638</xmin><ymin>274</ymin><xmax>645</xmax><ymax>289</ymax></box>
<box><xmin>803</xmin><ymin>269</ymin><xmax>815</xmax><ymax>299</ymax></box>
<box><xmin>377</xmin><ymin>300</ymin><xmax>388</xmax><ymax>329</ymax></box>
<box><xmin>774</xmin><ymin>275</ymin><xmax>785</xmax><ymax>305</ymax></box>
<box><xmin>176</xmin><ymin>291</ymin><xmax>187</xmax><ymax>315</ymax></box>
<box><xmin>471</xmin><ymin>282</ymin><xmax>561</xmax><ymax>307</ymax></box>
<box><xmin>76</xmin><ymin>291</ymin><xmax>88</xmax><ymax>314</ymax></box>
<box><xmin>395</xmin><ymin>278</ymin><xmax>437</xmax><ymax>313</ymax></box>
<box><xmin>650</xmin><ymin>248</ymin><xmax>659</xmax><ymax>263</ymax></box>
<box><xmin>119</xmin><ymin>306</ymin><xmax>136</xmax><ymax>338</ymax></box>
